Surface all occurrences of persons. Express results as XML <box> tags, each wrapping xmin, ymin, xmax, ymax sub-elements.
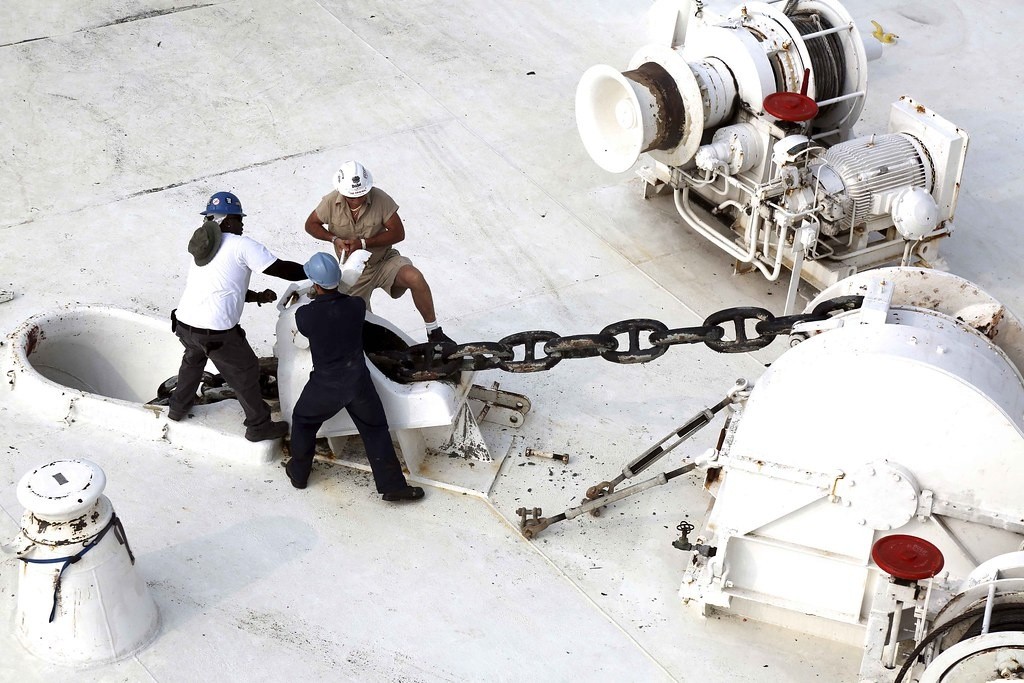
<box><xmin>283</xmin><ymin>250</ymin><xmax>429</xmax><ymax>506</ymax></box>
<box><xmin>306</xmin><ymin>159</ymin><xmax>461</xmax><ymax>354</ymax></box>
<box><xmin>167</xmin><ymin>191</ymin><xmax>306</xmax><ymax>443</ymax></box>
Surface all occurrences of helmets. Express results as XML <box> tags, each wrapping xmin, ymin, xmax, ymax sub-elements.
<box><xmin>199</xmin><ymin>192</ymin><xmax>245</xmax><ymax>216</ymax></box>
<box><xmin>331</xmin><ymin>160</ymin><xmax>372</xmax><ymax>198</ymax></box>
<box><xmin>303</xmin><ymin>251</ymin><xmax>341</xmax><ymax>290</ymax></box>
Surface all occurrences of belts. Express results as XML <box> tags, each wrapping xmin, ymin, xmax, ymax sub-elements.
<box><xmin>177</xmin><ymin>322</ymin><xmax>229</xmax><ymax>334</ymax></box>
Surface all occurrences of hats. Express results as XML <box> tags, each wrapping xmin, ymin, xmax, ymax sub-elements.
<box><xmin>187</xmin><ymin>222</ymin><xmax>223</xmax><ymax>266</ymax></box>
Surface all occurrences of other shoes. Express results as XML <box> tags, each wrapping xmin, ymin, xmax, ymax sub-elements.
<box><xmin>244</xmin><ymin>421</ymin><xmax>289</xmax><ymax>442</ymax></box>
<box><xmin>382</xmin><ymin>486</ymin><xmax>424</xmax><ymax>502</ymax></box>
<box><xmin>168</xmin><ymin>409</ymin><xmax>187</xmax><ymax>421</ymax></box>
<box><xmin>427</xmin><ymin>327</ymin><xmax>455</xmax><ymax>345</ymax></box>
<box><xmin>286</xmin><ymin>458</ymin><xmax>307</xmax><ymax>488</ymax></box>
